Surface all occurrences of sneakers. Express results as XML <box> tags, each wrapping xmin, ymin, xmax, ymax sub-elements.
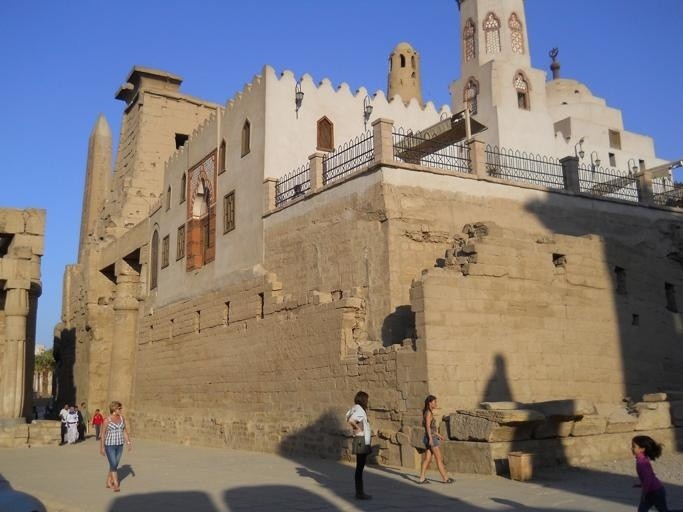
<box><xmin>441</xmin><ymin>478</ymin><xmax>456</xmax><ymax>483</ymax></box>
<box><xmin>419</xmin><ymin>478</ymin><xmax>431</xmax><ymax>484</ymax></box>
<box><xmin>357</xmin><ymin>493</ymin><xmax>373</xmax><ymax>500</ymax></box>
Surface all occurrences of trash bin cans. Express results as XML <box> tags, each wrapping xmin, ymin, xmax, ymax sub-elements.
<box><xmin>560</xmin><ymin>156</ymin><xmax>580</xmax><ymax>192</ymax></box>
<box><xmin>507</xmin><ymin>452</ymin><xmax>535</xmax><ymax>482</ymax></box>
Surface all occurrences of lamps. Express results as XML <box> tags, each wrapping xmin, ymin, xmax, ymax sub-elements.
<box><xmin>574</xmin><ymin>136</ymin><xmax>585</xmax><ymax>161</ymax></box>
<box><xmin>590</xmin><ymin>150</ymin><xmax>600</xmax><ymax>174</ymax></box>
<box><xmin>362</xmin><ymin>94</ymin><xmax>373</xmax><ymax>134</ymax></box>
<box><xmin>627</xmin><ymin>158</ymin><xmax>638</xmax><ymax>175</ymax></box>
<box><xmin>294</xmin><ymin>78</ymin><xmax>303</xmax><ymax>119</ymax></box>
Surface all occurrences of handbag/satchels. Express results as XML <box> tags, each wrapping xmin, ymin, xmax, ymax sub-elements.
<box><xmin>352</xmin><ymin>435</ymin><xmax>367</xmax><ymax>455</ymax></box>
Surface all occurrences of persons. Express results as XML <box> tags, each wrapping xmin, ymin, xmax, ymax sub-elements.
<box><xmin>418</xmin><ymin>393</ymin><xmax>453</xmax><ymax>485</ymax></box>
<box><xmin>630</xmin><ymin>434</ymin><xmax>667</xmax><ymax>511</ymax></box>
<box><xmin>91</xmin><ymin>408</ymin><xmax>103</xmax><ymax>441</ymax></box>
<box><xmin>56</xmin><ymin>401</ymin><xmax>89</xmax><ymax>446</ymax></box>
<box><xmin>345</xmin><ymin>390</ymin><xmax>374</xmax><ymax>500</ymax></box>
<box><xmin>99</xmin><ymin>401</ymin><xmax>129</xmax><ymax>493</ymax></box>
